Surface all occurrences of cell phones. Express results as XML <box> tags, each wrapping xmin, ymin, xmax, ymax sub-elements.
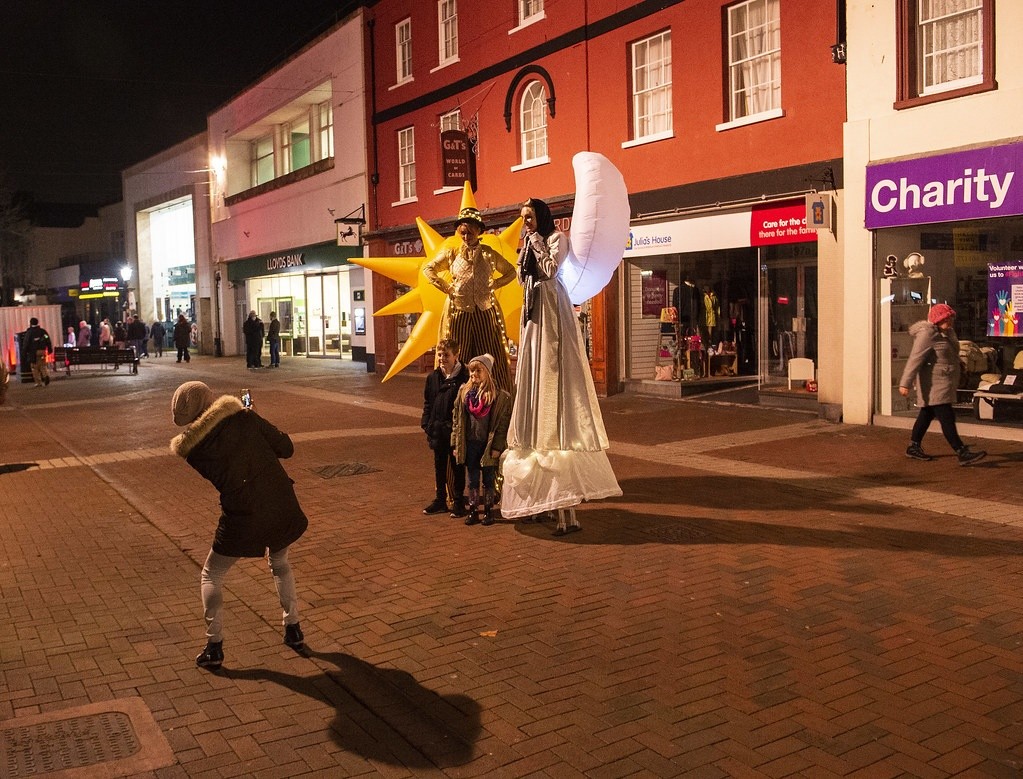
<box><xmin>241</xmin><ymin>389</ymin><xmax>252</xmax><ymax>408</ymax></box>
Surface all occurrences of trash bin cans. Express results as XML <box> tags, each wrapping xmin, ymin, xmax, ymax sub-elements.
<box><xmin>13</xmin><ymin>332</ymin><xmax>34</xmax><ymax>382</ymax></box>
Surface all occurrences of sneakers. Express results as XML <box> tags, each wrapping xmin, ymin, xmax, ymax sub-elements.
<box><xmin>450</xmin><ymin>504</ymin><xmax>465</xmax><ymax>518</ymax></box>
<box><xmin>282</xmin><ymin>620</ymin><xmax>304</xmax><ymax>645</ymax></box>
<box><xmin>196</xmin><ymin>641</ymin><xmax>223</xmax><ymax>665</ymax></box>
<box><xmin>423</xmin><ymin>500</ymin><xmax>448</xmax><ymax>515</ymax></box>
<box><xmin>957</xmin><ymin>449</ymin><xmax>986</xmax><ymax>466</ymax></box>
<box><xmin>906</xmin><ymin>442</ymin><xmax>931</xmax><ymax>461</ymax></box>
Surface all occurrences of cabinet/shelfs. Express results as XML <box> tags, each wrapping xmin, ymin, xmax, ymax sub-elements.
<box><xmin>881</xmin><ymin>278</ymin><xmax>932</xmax><ymax>416</ymax></box>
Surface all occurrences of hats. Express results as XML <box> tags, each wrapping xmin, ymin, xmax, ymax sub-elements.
<box><xmin>170</xmin><ymin>381</ymin><xmax>212</xmax><ymax>426</ymax></box>
<box><xmin>454</xmin><ymin>209</ymin><xmax>485</xmax><ymax>235</ymax></box>
<box><xmin>471</xmin><ymin>353</ymin><xmax>495</xmax><ymax>374</ymax></box>
<box><xmin>929</xmin><ymin>305</ymin><xmax>955</xmax><ymax>324</ymax></box>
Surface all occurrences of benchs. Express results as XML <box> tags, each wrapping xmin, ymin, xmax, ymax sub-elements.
<box><xmin>54</xmin><ymin>347</ymin><xmax>138</xmax><ymax>375</ymax></box>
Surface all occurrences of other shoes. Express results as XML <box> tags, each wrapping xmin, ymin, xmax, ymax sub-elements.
<box><xmin>35</xmin><ymin>383</ymin><xmax>42</xmax><ymax>388</ymax></box>
<box><xmin>45</xmin><ymin>379</ymin><xmax>50</xmax><ymax>386</ymax></box>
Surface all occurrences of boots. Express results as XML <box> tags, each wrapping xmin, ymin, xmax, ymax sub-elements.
<box><xmin>465</xmin><ymin>486</ymin><xmax>481</xmax><ymax>525</ymax></box>
<box><xmin>480</xmin><ymin>487</ymin><xmax>496</xmax><ymax>525</ymax></box>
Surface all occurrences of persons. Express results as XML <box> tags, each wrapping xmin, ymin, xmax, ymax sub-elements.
<box><xmin>449</xmin><ymin>355</ymin><xmax>511</xmax><ymax>525</ymax></box>
<box><xmin>516</xmin><ymin>198</ymin><xmax>587</xmax><ymax>374</ymax></box>
<box><xmin>170</xmin><ymin>380</ymin><xmax>309</xmax><ymax>667</ymax></box>
<box><xmin>266</xmin><ymin>312</ymin><xmax>280</xmax><ymax>367</ymax></box>
<box><xmin>243</xmin><ymin>311</ymin><xmax>265</xmax><ymax>369</ymax></box>
<box><xmin>149</xmin><ymin>319</ymin><xmax>166</xmax><ymax>357</ymax></box>
<box><xmin>23</xmin><ymin>318</ymin><xmax>52</xmax><ymax>387</ymax></box>
<box><xmin>898</xmin><ymin>304</ymin><xmax>987</xmax><ymax>466</ymax></box>
<box><xmin>420</xmin><ymin>339</ymin><xmax>468</xmax><ymax>518</ymax></box>
<box><xmin>67</xmin><ymin>314</ymin><xmax>150</xmax><ymax>358</ymax></box>
<box><xmin>173</xmin><ymin>315</ymin><xmax>191</xmax><ymax>363</ymax></box>
<box><xmin>424</xmin><ymin>219</ymin><xmax>511</xmax><ymax>380</ymax></box>
